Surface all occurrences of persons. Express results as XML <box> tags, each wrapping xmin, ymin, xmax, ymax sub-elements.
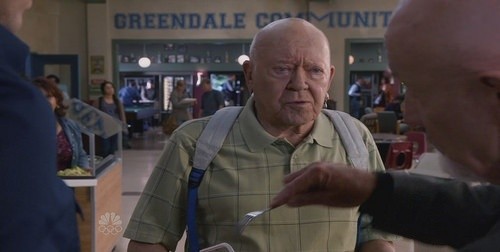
<box><xmin>0</xmin><ymin>0</ymin><xmax>243</xmax><ymax>252</ymax></box>
<box><xmin>271</xmin><ymin>0</ymin><xmax>500</xmax><ymax>252</ymax></box>
<box><xmin>121</xmin><ymin>17</ymin><xmax>401</xmax><ymax>252</ymax></box>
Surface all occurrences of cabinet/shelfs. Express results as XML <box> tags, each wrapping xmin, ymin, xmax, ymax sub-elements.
<box><xmin>57</xmin><ymin>98</ymin><xmax>125</xmax><ymax>252</ymax></box>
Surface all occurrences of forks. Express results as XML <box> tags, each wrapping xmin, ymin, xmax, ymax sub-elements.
<box><xmin>238</xmin><ymin>203</ymin><xmax>287</xmax><ymax>233</ymax></box>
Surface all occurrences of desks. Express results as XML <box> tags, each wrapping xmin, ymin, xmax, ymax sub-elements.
<box><xmin>372</xmin><ymin>132</ymin><xmax>406</xmax><ymax>144</ymax></box>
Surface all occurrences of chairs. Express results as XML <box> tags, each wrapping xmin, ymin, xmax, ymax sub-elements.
<box><xmin>384</xmin><ymin>131</ymin><xmax>427</xmax><ymax>171</ymax></box>
